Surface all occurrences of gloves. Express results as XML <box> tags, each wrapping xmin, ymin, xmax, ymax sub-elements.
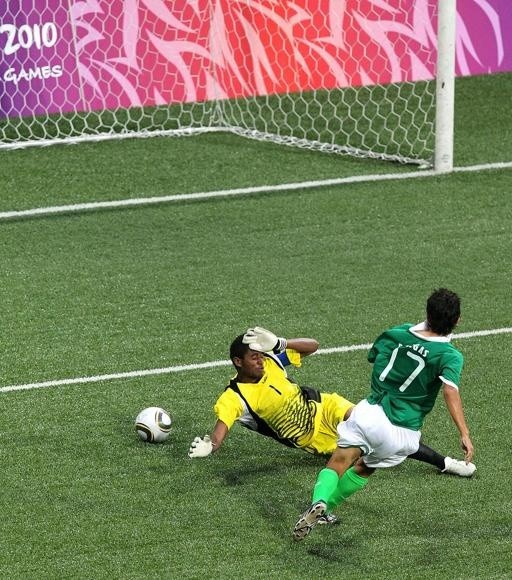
<box><xmin>242</xmin><ymin>326</ymin><xmax>281</xmax><ymax>355</ymax></box>
<box><xmin>187</xmin><ymin>434</ymin><xmax>213</xmax><ymax>458</ymax></box>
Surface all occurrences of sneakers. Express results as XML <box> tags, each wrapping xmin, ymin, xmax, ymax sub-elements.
<box><xmin>292</xmin><ymin>500</ymin><xmax>339</xmax><ymax>542</ymax></box>
<box><xmin>437</xmin><ymin>457</ymin><xmax>477</xmax><ymax>478</ymax></box>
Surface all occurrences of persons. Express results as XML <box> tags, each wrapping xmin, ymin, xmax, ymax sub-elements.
<box><xmin>291</xmin><ymin>286</ymin><xmax>465</xmax><ymax>546</ymax></box>
<box><xmin>184</xmin><ymin>325</ymin><xmax>477</xmax><ymax>480</ymax></box>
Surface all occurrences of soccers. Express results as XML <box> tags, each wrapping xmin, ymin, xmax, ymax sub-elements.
<box><xmin>135</xmin><ymin>407</ymin><xmax>172</xmax><ymax>444</ymax></box>
